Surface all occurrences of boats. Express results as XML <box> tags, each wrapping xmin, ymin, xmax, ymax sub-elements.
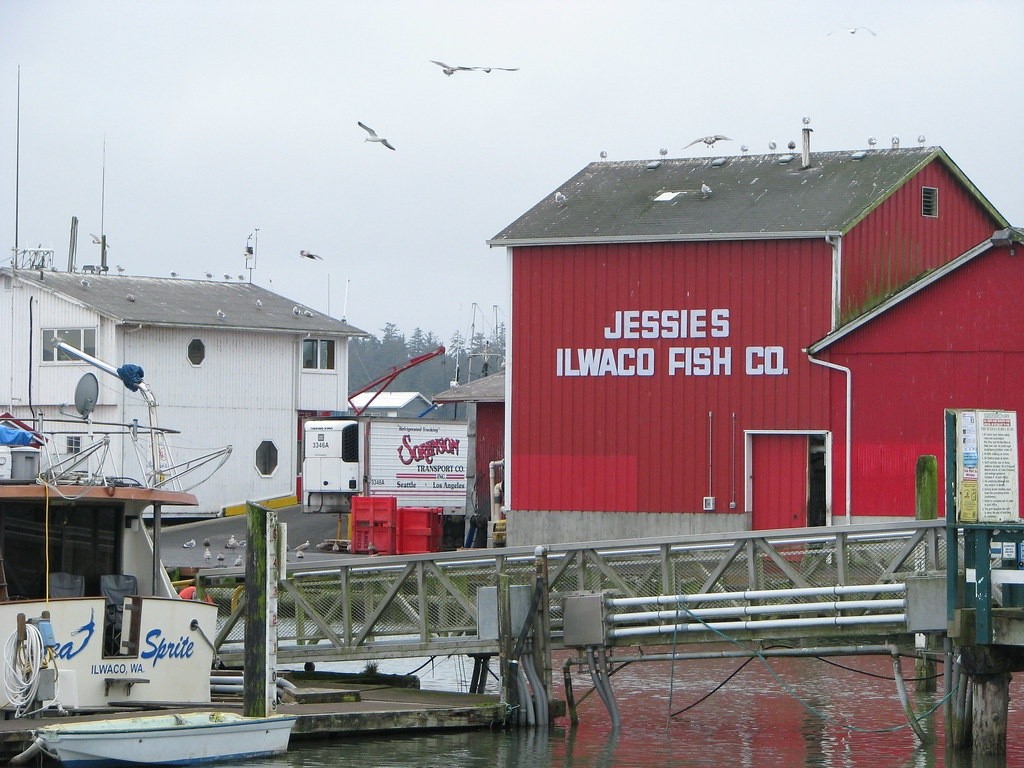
<box><xmin>0</xmin><ymin>335</ymin><xmax>220</xmax><ymax>714</ymax></box>
<box><xmin>32</xmin><ymin>711</ymin><xmax>298</xmax><ymax>768</ymax></box>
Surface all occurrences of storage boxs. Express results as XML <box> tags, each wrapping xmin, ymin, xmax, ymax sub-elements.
<box><xmin>351</xmin><ymin>495</ymin><xmax>397</xmax><ymax>555</ymax></box>
<box><xmin>395</xmin><ymin>506</ymin><xmax>444</xmax><ymax>554</ymax></box>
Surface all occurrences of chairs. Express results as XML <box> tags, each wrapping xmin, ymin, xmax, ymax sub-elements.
<box><xmin>98</xmin><ymin>573</ymin><xmax>140</xmax><ymax>655</ymax></box>
<box><xmin>48</xmin><ymin>573</ymin><xmax>85</xmax><ymax>597</ymax></box>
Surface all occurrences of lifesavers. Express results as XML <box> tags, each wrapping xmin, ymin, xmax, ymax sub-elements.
<box><xmin>178</xmin><ymin>586</ymin><xmax>213</xmax><ymax>603</ymax></box>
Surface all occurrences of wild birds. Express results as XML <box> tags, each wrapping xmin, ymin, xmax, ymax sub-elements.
<box><xmin>918</xmin><ymin>135</ymin><xmax>925</xmax><ymax>147</ymax></box>
<box><xmin>892</xmin><ymin>137</ymin><xmax>899</xmax><ymax>149</ymax></box>
<box><xmin>299</xmin><ymin>249</ymin><xmax>324</xmax><ymax>262</ymax></box>
<box><xmin>553</xmin><ymin>191</ymin><xmax>568</xmax><ymax>208</ymax></box>
<box><xmin>234</xmin><ymin>555</ymin><xmax>243</xmax><ymax>569</ymax></box>
<box><xmin>223</xmin><ymin>273</ymin><xmax>246</xmax><ymax>284</ymax></box>
<box><xmin>357</xmin><ymin>120</ymin><xmax>396</xmax><ymax>151</ymax></box>
<box><xmin>600</xmin><ymin>151</ymin><xmax>608</xmax><ymax>163</ymax></box>
<box><xmin>79</xmin><ymin>278</ymin><xmax>92</xmax><ymax>291</ymax></box>
<box><xmin>741</xmin><ymin>145</ymin><xmax>749</xmax><ymax>157</ymax></box>
<box><xmin>202</xmin><ymin>538</ymin><xmax>212</xmax><ymax>565</ymax></box>
<box><xmin>204</xmin><ymin>270</ymin><xmax>215</xmax><ymax>282</ymax></box>
<box><xmin>429</xmin><ymin>59</ymin><xmax>519</xmax><ymax>76</ymax></box>
<box><xmin>169</xmin><ymin>270</ymin><xmax>180</xmax><ymax>281</ymax></box>
<box><xmin>216</xmin><ymin>309</ymin><xmax>227</xmax><ymax>322</ymax></box>
<box><xmin>291</xmin><ymin>305</ymin><xmax>314</xmax><ymax>318</ymax></box>
<box><xmin>681</xmin><ymin>134</ymin><xmax>735</xmax><ymax>149</ymax></box>
<box><xmin>216</xmin><ymin>553</ymin><xmax>225</xmax><ymax>568</ymax></box>
<box><xmin>367</xmin><ymin>541</ymin><xmax>382</xmax><ymax>557</ymax></box>
<box><xmin>701</xmin><ymin>183</ymin><xmax>713</xmax><ymax>199</ymax></box>
<box><xmin>660</xmin><ymin>149</ymin><xmax>667</xmax><ymax>158</ymax></box>
<box><xmin>788</xmin><ymin>141</ymin><xmax>796</xmax><ymax>155</ymax></box>
<box><xmin>182</xmin><ymin>537</ymin><xmax>197</xmax><ymax>552</ymax></box>
<box><xmin>94</xmin><ymin>265</ymin><xmax>101</xmax><ymax>278</ymax></box>
<box><xmin>50</xmin><ymin>265</ymin><xmax>58</xmax><ymax>273</ymax></box>
<box><xmin>254</xmin><ymin>299</ymin><xmax>263</xmax><ymax>312</ymax></box>
<box><xmin>868</xmin><ymin>137</ymin><xmax>876</xmax><ymax>149</ymax></box>
<box><xmin>294</xmin><ymin>539</ymin><xmax>311</xmax><ymax>563</ymax></box>
<box><xmin>316</xmin><ymin>542</ymin><xmax>328</xmax><ymax>554</ymax></box>
<box><xmin>126</xmin><ymin>293</ymin><xmax>135</xmax><ymax>303</ymax></box>
<box><xmin>769</xmin><ymin>140</ymin><xmax>777</xmax><ymax>154</ymax></box>
<box><xmin>332</xmin><ymin>541</ymin><xmax>340</xmax><ymax>555</ymax></box>
<box><xmin>226</xmin><ymin>534</ymin><xmax>246</xmax><ymax>554</ymax></box>
<box><xmin>825</xmin><ymin>25</ymin><xmax>877</xmax><ymax>38</ymax></box>
<box><xmin>116</xmin><ymin>264</ymin><xmax>125</xmax><ymax>275</ymax></box>
<box><xmin>345</xmin><ymin>542</ymin><xmax>353</xmax><ymax>555</ymax></box>
